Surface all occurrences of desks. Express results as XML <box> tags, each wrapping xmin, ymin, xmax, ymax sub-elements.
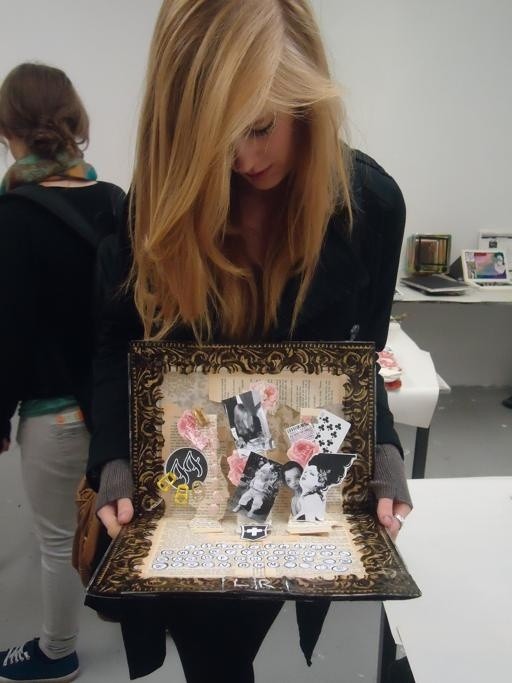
<box><xmin>370</xmin><ymin>314</ymin><xmax>452</xmax><ymax>475</ymax></box>
<box><xmin>392</xmin><ymin>282</ymin><xmax>509</xmax><ymax>304</ymax></box>
<box><xmin>369</xmin><ymin>477</ymin><xmax>509</xmax><ymax>681</ymax></box>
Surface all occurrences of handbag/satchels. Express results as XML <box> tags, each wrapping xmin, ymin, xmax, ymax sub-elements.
<box><xmin>70</xmin><ymin>474</ymin><xmax>127</xmax><ymax>625</ymax></box>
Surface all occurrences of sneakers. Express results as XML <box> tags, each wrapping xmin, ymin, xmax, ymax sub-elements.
<box><xmin>0</xmin><ymin>636</ymin><xmax>81</xmax><ymax>682</ymax></box>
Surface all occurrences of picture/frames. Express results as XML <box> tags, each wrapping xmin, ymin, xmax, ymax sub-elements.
<box><xmin>460</xmin><ymin>247</ymin><xmax>509</xmax><ymax>284</ymax></box>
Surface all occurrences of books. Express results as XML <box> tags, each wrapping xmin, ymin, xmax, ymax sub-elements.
<box><xmin>399</xmin><ymin>273</ymin><xmax>471</xmax><ymax>293</ymax></box>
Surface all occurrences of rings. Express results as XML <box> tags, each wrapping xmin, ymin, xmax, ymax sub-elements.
<box><xmin>395</xmin><ymin>513</ymin><xmax>407</xmax><ymax>529</ymax></box>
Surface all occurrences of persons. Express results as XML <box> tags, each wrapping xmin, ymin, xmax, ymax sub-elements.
<box><xmin>83</xmin><ymin>2</ymin><xmax>407</xmax><ymax>681</ymax></box>
<box><xmin>280</xmin><ymin>462</ymin><xmax>303</xmax><ymax>518</ymax></box>
<box><xmin>231</xmin><ymin>464</ymin><xmax>276</xmax><ymax>517</ymax></box>
<box><xmin>0</xmin><ymin>62</ymin><xmax>126</xmax><ymax>682</ymax></box>
<box><xmin>231</xmin><ymin>404</ymin><xmax>263</xmax><ymax>448</ymax></box>
<box><xmin>490</xmin><ymin>253</ymin><xmax>505</xmax><ymax>278</ymax></box>
<box><xmin>297</xmin><ymin>454</ymin><xmax>354</xmax><ymax>522</ymax></box>
